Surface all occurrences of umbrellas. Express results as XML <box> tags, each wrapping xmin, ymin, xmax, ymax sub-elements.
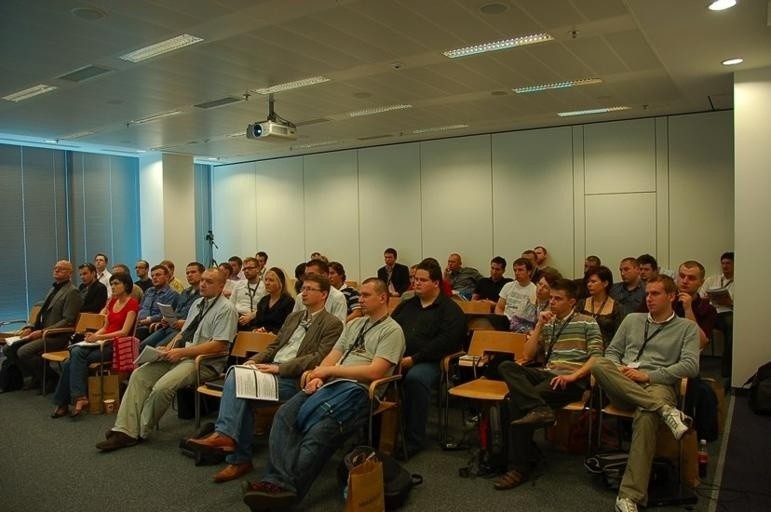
<box><xmin>511</xmin><ymin>407</ymin><xmax>556</xmax><ymax>428</ymax></box>
<box><xmin>187</xmin><ymin>433</ymin><xmax>235</xmax><ymax>452</ymax></box>
<box><xmin>70</xmin><ymin>398</ymin><xmax>89</xmax><ymax>417</ymax></box>
<box><xmin>23</xmin><ymin>383</ymin><xmax>35</xmax><ymax>391</ymax></box>
<box><xmin>494</xmin><ymin>470</ymin><xmax>528</xmax><ymax>489</ymax></box>
<box><xmin>50</xmin><ymin>405</ymin><xmax>69</xmax><ymax>419</ymax></box>
<box><xmin>213</xmin><ymin>461</ymin><xmax>254</xmax><ymax>483</ymax></box>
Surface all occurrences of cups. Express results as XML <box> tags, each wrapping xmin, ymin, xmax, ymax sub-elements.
<box><xmin>301</xmin><ymin>287</ymin><xmax>320</xmax><ymax>295</ymax></box>
<box><xmin>242</xmin><ymin>266</ymin><xmax>256</xmax><ymax>271</ymax></box>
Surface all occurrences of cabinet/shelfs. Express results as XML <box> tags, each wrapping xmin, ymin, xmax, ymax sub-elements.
<box><xmin>697</xmin><ymin>439</ymin><xmax>708</xmax><ymax>479</ymax></box>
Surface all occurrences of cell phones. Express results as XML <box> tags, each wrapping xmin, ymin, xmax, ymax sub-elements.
<box><xmin>206</xmin><ymin>229</ymin><xmax>218</xmax><ymax>250</ymax></box>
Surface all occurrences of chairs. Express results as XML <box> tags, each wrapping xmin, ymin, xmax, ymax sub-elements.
<box><xmin>429</xmin><ymin>301</ymin><xmax>723</xmax><ymax>503</ymax></box>
<box><xmin>96</xmin><ymin>430</ymin><xmax>145</xmax><ymax>451</ymax></box>
<box><xmin>242</xmin><ymin>479</ymin><xmax>298</xmax><ymax>512</ymax></box>
<box><xmin>0</xmin><ymin>281</ymin><xmax>402</xmax><ymax>462</ymax></box>
<box><xmin>614</xmin><ymin>496</ymin><xmax>637</xmax><ymax>512</ymax></box>
<box><xmin>661</xmin><ymin>405</ymin><xmax>693</xmax><ymax>442</ymax></box>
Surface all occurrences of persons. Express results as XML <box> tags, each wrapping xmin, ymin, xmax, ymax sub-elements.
<box><xmin>534</xmin><ymin>247</ymin><xmax>547</xmax><ymax>271</ymax></box>
<box><xmin>185</xmin><ymin>271</ymin><xmax>344</xmax><ymax>480</ymax></box>
<box><xmin>699</xmin><ymin>252</ymin><xmax>734</xmax><ymax>376</ymax></box>
<box><xmin>592</xmin><ymin>274</ymin><xmax>701</xmax><ymax>512</ymax></box>
<box><xmin>134</xmin><ymin>260</ymin><xmax>153</xmax><ymax>293</ymax></box>
<box><xmin>241</xmin><ymin>278</ymin><xmax>404</xmax><ymax>511</ymax></box>
<box><xmin>292</xmin><ymin>259</ymin><xmax>347</xmax><ymax>328</ymax></box>
<box><xmin>248</xmin><ymin>267</ymin><xmax>295</xmax><ymax>334</ymax></box>
<box><xmin>96</xmin><ymin>269</ymin><xmax>240</xmax><ymax>450</ymax></box>
<box><xmin>639</xmin><ymin>255</ymin><xmax>657</xmax><ymax>283</ymax></box>
<box><xmin>139</xmin><ymin>260</ymin><xmax>204</xmax><ymax>352</ymax></box>
<box><xmin>377</xmin><ymin>248</ymin><xmax>410</xmax><ymax>298</ymax></box>
<box><xmin>610</xmin><ymin>258</ymin><xmax>649</xmax><ymax>317</ymax></box>
<box><xmin>522</xmin><ymin>250</ymin><xmax>539</xmax><ymax>278</ymax></box>
<box><xmin>583</xmin><ymin>256</ymin><xmax>600</xmax><ymax>275</ymax></box>
<box><xmin>95</xmin><ymin>254</ymin><xmax>112</xmax><ymax>299</ymax></box>
<box><xmin>444</xmin><ymin>253</ymin><xmax>483</xmax><ymax>299</ymax></box>
<box><xmin>228</xmin><ymin>256</ymin><xmax>242</xmax><ymax>280</ymax></box>
<box><xmin>672</xmin><ymin>260</ymin><xmax>717</xmax><ymax>350</ymax></box>
<box><xmin>232</xmin><ymin>257</ymin><xmax>268</xmax><ymax>331</ymax></box>
<box><xmin>327</xmin><ymin>261</ymin><xmax>363</xmax><ymax>322</ymax></box>
<box><xmin>3</xmin><ymin>260</ymin><xmax>80</xmax><ymax>392</ymax></box>
<box><xmin>112</xmin><ymin>264</ymin><xmax>143</xmax><ymax>299</ymax></box>
<box><xmin>219</xmin><ymin>263</ymin><xmax>235</xmax><ymax>298</ymax></box>
<box><xmin>577</xmin><ymin>267</ymin><xmax>624</xmax><ymax>343</ymax></box>
<box><xmin>50</xmin><ymin>271</ymin><xmax>138</xmax><ymax>418</ymax></box>
<box><xmin>134</xmin><ymin>265</ymin><xmax>179</xmax><ymax>341</ymax></box>
<box><xmin>385</xmin><ymin>257</ymin><xmax>467</xmax><ymax>457</ymax></box>
<box><xmin>509</xmin><ymin>269</ymin><xmax>560</xmax><ymax>335</ymax></box>
<box><xmin>472</xmin><ymin>256</ymin><xmax>513</xmax><ymax>302</ymax></box>
<box><xmin>161</xmin><ymin>259</ymin><xmax>183</xmax><ymax>294</ymax></box>
<box><xmin>78</xmin><ymin>263</ymin><xmax>107</xmax><ymax>313</ymax></box>
<box><xmin>494</xmin><ymin>280</ymin><xmax>604</xmax><ymax>493</ymax></box>
<box><xmin>493</xmin><ymin>257</ymin><xmax>539</xmax><ymax>322</ymax></box>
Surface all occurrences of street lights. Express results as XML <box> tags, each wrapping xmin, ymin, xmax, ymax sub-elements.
<box><xmin>0</xmin><ymin>359</ymin><xmax>24</xmax><ymax>392</ymax></box>
<box><xmin>341</xmin><ymin>441</ymin><xmax>416</xmax><ymax>512</ymax></box>
<box><xmin>749</xmin><ymin>361</ymin><xmax>771</xmax><ymax>418</ymax></box>
<box><xmin>87</xmin><ymin>370</ymin><xmax>119</xmax><ymax>415</ymax></box>
<box><xmin>584</xmin><ymin>451</ymin><xmax>628</xmax><ymax>490</ymax></box>
<box><xmin>452</xmin><ymin>425</ymin><xmax>483</xmax><ymax>447</ymax></box>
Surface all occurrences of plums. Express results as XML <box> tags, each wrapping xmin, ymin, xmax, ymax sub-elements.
<box><xmin>246</xmin><ymin>120</ymin><xmax>298</xmax><ymax>144</ymax></box>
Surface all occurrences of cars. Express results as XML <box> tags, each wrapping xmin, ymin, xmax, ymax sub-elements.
<box><xmin>103</xmin><ymin>399</ymin><xmax>115</xmax><ymax>414</ymax></box>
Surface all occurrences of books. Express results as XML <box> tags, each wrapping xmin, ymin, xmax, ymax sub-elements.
<box><xmin>225</xmin><ymin>365</ymin><xmax>280</xmax><ymax>401</ymax></box>
<box><xmin>5</xmin><ymin>336</ymin><xmax>30</xmax><ymax>346</ymax></box>
<box><xmin>157</xmin><ymin>303</ymin><xmax>176</xmax><ymax>323</ymax></box>
<box><xmin>133</xmin><ymin>345</ymin><xmax>168</xmax><ymax>362</ymax></box>
<box><xmin>68</xmin><ymin>340</ymin><xmax>106</xmax><ymax>348</ymax></box>
